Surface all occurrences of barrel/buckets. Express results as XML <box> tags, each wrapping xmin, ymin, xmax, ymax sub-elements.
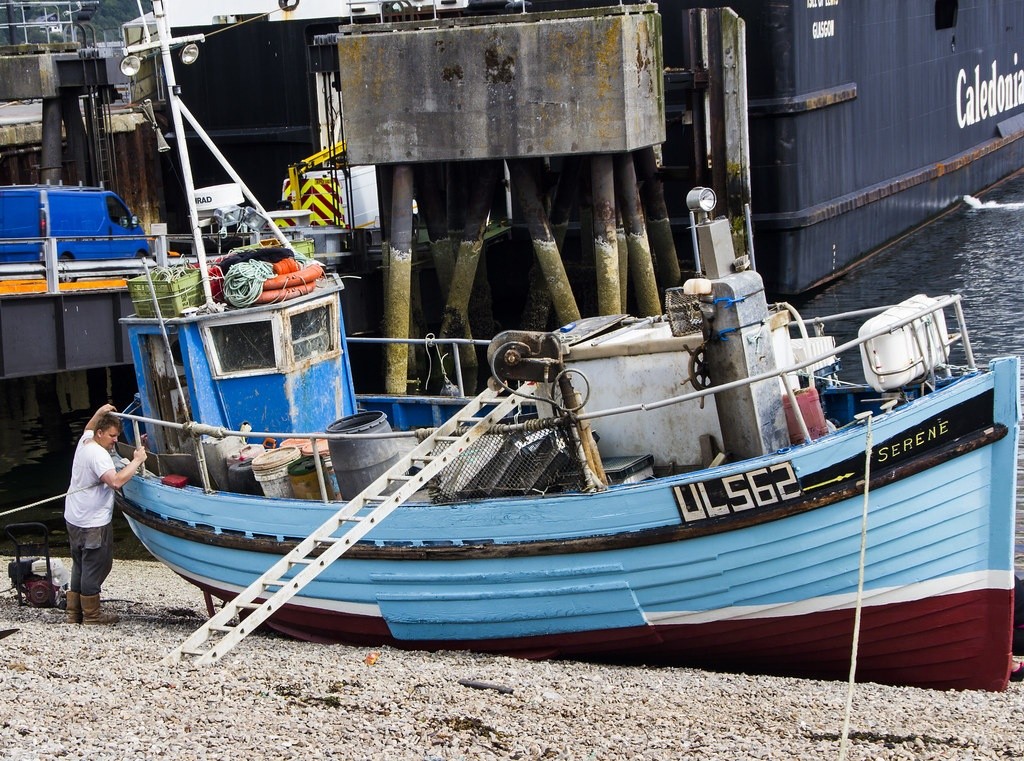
<box><xmin>783</xmin><ymin>387</ymin><xmax>829</xmax><ymax>446</ymax></box>
<box><xmin>226</xmin><ymin>411</ymin><xmax>409</xmax><ymax>501</ymax></box>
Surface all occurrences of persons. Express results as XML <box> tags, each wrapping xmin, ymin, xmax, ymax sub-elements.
<box><xmin>63</xmin><ymin>403</ymin><xmax>149</xmax><ymax>626</ymax></box>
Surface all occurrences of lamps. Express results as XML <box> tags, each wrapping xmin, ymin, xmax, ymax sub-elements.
<box><xmin>180</xmin><ymin>44</ymin><xmax>199</xmax><ymax>66</ymax></box>
<box><xmin>117</xmin><ymin>56</ymin><xmax>143</xmax><ymax>77</ymax></box>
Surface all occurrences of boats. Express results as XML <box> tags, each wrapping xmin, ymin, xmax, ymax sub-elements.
<box><xmin>111</xmin><ymin>0</ymin><xmax>1020</xmax><ymax>691</ymax></box>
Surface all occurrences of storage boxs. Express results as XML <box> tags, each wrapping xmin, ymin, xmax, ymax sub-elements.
<box><xmin>126</xmin><ymin>256</ymin><xmax>224</xmax><ymax>319</ymax></box>
<box><xmin>456</xmin><ymin>431</ymin><xmax>571</xmax><ymax>499</ymax></box>
<box><xmin>233</xmin><ymin>239</ymin><xmax>316</xmax><ymax>260</ymax></box>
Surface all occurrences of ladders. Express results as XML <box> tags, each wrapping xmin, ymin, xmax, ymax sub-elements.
<box><xmin>158</xmin><ymin>375</ymin><xmax>539</xmax><ymax>678</ymax></box>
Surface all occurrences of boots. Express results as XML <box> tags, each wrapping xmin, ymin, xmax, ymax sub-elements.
<box><xmin>66</xmin><ymin>591</ymin><xmax>83</xmax><ymax>623</ymax></box>
<box><xmin>80</xmin><ymin>594</ymin><xmax>119</xmax><ymax>625</ymax></box>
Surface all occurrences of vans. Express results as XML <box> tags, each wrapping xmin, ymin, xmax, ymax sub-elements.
<box><xmin>0</xmin><ymin>180</ymin><xmax>156</xmax><ymax>283</ymax></box>
